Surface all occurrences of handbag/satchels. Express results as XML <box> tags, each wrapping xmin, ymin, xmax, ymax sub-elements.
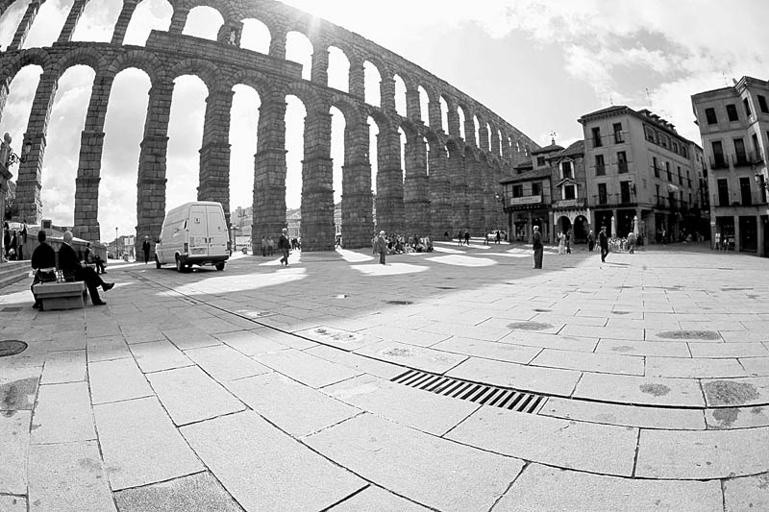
<box><xmin>36</xmin><ymin>270</ymin><xmax>56</xmax><ymax>282</ymax></box>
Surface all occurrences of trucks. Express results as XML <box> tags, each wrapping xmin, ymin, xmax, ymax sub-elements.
<box><xmin>154</xmin><ymin>200</ymin><xmax>234</xmax><ymax>273</ymax></box>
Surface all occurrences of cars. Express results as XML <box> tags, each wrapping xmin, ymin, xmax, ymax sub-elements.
<box><xmin>487</xmin><ymin>229</ymin><xmax>507</xmax><ymax>241</ymax></box>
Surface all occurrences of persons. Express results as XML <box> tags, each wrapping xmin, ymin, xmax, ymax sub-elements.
<box><xmin>84</xmin><ymin>243</ymin><xmax>107</xmax><ymax>274</ymax></box>
<box><xmin>143</xmin><ymin>239</ymin><xmax>150</xmax><ymax>263</ymax></box>
<box><xmin>59</xmin><ymin>233</ymin><xmax>116</xmax><ymax>305</ymax></box>
<box><xmin>457</xmin><ymin>225</ymin><xmax>729</xmax><ymax>269</ymax></box>
<box><xmin>31</xmin><ymin>231</ymin><xmax>57</xmax><ymax>311</ymax></box>
<box><xmin>4</xmin><ymin>230</ymin><xmax>25</xmax><ymax>261</ymax></box>
<box><xmin>371</xmin><ymin>231</ymin><xmax>433</xmax><ymax>264</ymax></box>
<box><xmin>261</xmin><ymin>228</ymin><xmax>300</xmax><ymax>266</ymax></box>
<box><xmin>336</xmin><ymin>235</ymin><xmax>342</xmax><ymax>247</ymax></box>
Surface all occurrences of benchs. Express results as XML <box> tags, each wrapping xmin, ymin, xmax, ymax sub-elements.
<box><xmin>33</xmin><ymin>279</ymin><xmax>87</xmax><ymax>311</ymax></box>
<box><xmin>80</xmin><ymin>259</ymin><xmax>96</xmax><ymax>272</ymax></box>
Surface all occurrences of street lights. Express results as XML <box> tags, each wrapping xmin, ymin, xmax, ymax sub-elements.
<box><xmin>115</xmin><ymin>226</ymin><xmax>118</xmax><ymax>259</ymax></box>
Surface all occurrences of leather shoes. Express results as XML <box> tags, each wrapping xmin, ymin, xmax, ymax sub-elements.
<box><xmin>103</xmin><ymin>283</ymin><xmax>114</xmax><ymax>291</ymax></box>
<box><xmin>95</xmin><ymin>301</ymin><xmax>106</xmax><ymax>305</ymax></box>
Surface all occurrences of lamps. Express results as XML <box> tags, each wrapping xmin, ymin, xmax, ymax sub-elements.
<box><xmin>754</xmin><ymin>171</ymin><xmax>769</xmax><ymax>192</ymax></box>
<box><xmin>5</xmin><ymin>137</ymin><xmax>32</xmax><ymax>167</ymax></box>
